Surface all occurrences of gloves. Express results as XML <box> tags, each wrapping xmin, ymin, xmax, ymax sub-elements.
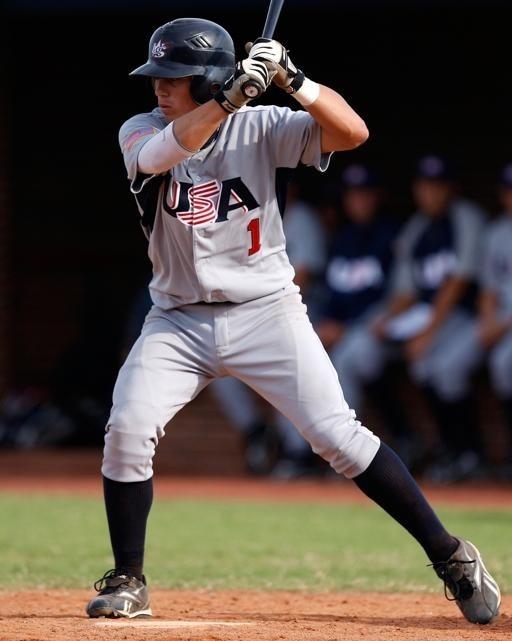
<box><xmin>216</xmin><ymin>58</ymin><xmax>275</xmax><ymax>115</ymax></box>
<box><xmin>249</xmin><ymin>37</ymin><xmax>304</xmax><ymax>98</ymax></box>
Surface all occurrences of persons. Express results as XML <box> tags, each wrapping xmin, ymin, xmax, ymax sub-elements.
<box><xmin>209</xmin><ymin>154</ymin><xmax>511</xmax><ymax>479</ymax></box>
<box><xmin>87</xmin><ymin>17</ymin><xmax>502</xmax><ymax>624</ymax></box>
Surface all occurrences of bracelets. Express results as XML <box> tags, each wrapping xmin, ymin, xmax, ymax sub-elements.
<box><xmin>289</xmin><ymin>76</ymin><xmax>320</xmax><ymax>106</ymax></box>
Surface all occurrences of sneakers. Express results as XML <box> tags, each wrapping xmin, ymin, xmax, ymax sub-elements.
<box><xmin>433</xmin><ymin>537</ymin><xmax>500</xmax><ymax>624</ymax></box>
<box><xmin>87</xmin><ymin>573</ymin><xmax>151</xmax><ymax>618</ymax></box>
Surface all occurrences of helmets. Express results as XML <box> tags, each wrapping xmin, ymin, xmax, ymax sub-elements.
<box><xmin>131</xmin><ymin>18</ymin><xmax>236</xmax><ymax>103</ymax></box>
